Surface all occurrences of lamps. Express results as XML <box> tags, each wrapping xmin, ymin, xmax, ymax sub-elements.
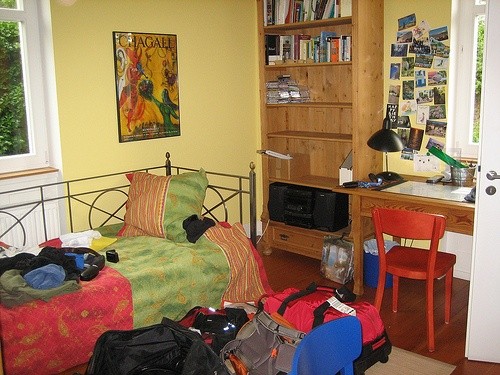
<box><xmin>367</xmin><ymin>119</ymin><xmax>404</xmax><ymax>183</ymax></box>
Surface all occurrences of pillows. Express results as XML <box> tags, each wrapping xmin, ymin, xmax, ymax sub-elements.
<box><xmin>116</xmin><ymin>169</ymin><xmax>209</xmax><ymax>243</ymax></box>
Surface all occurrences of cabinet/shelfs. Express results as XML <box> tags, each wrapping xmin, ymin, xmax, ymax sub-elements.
<box><xmin>256</xmin><ymin>0</ymin><xmax>384</xmax><ymax>255</ymax></box>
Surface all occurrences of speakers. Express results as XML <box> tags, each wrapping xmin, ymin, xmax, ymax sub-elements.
<box><xmin>313</xmin><ymin>190</ymin><xmax>349</xmax><ymax>232</ymax></box>
<box><xmin>268</xmin><ymin>182</ymin><xmax>284</xmax><ymax>222</ymax></box>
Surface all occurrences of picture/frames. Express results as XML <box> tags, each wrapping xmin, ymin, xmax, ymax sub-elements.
<box><xmin>114</xmin><ymin>31</ymin><xmax>181</xmax><ymax>142</ymax></box>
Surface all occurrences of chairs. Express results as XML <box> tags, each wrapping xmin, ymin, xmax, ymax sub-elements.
<box><xmin>371</xmin><ymin>207</ymin><xmax>456</xmax><ymax>352</ymax></box>
<box><xmin>289</xmin><ymin>317</ymin><xmax>363</xmax><ymax>375</ymax></box>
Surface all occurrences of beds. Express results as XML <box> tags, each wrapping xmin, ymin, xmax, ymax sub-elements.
<box><xmin>0</xmin><ymin>152</ymin><xmax>275</xmax><ymax>375</ymax></box>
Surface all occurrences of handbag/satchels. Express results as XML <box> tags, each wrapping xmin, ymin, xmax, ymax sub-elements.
<box><xmin>221</xmin><ymin>311</ymin><xmax>308</xmax><ymax>375</ymax></box>
<box><xmin>319</xmin><ymin>233</ymin><xmax>355</xmax><ymax>285</ymax></box>
<box><xmin>254</xmin><ymin>281</ymin><xmax>392</xmax><ymax>375</ymax></box>
<box><xmin>85</xmin><ymin>307</ymin><xmax>250</xmax><ymax>375</ymax></box>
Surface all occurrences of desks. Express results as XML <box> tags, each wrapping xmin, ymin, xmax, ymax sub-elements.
<box><xmin>350</xmin><ymin>173</ymin><xmax>475</xmax><ymax>297</ymax></box>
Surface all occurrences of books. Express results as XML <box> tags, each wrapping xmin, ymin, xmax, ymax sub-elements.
<box><xmin>262</xmin><ymin>0</ymin><xmax>352</xmax><ymax>65</ymax></box>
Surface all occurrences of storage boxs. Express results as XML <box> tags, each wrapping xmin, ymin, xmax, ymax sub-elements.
<box><xmin>364</xmin><ymin>250</ymin><xmax>393</xmax><ymax>288</ymax></box>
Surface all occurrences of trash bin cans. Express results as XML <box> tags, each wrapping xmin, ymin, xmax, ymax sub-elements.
<box><xmin>363</xmin><ymin>239</ymin><xmax>402</xmax><ymax>288</ymax></box>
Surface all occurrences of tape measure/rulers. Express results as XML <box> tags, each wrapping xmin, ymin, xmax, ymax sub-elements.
<box><xmin>429</xmin><ymin>146</ymin><xmax>467</xmax><ymax>168</ymax></box>
